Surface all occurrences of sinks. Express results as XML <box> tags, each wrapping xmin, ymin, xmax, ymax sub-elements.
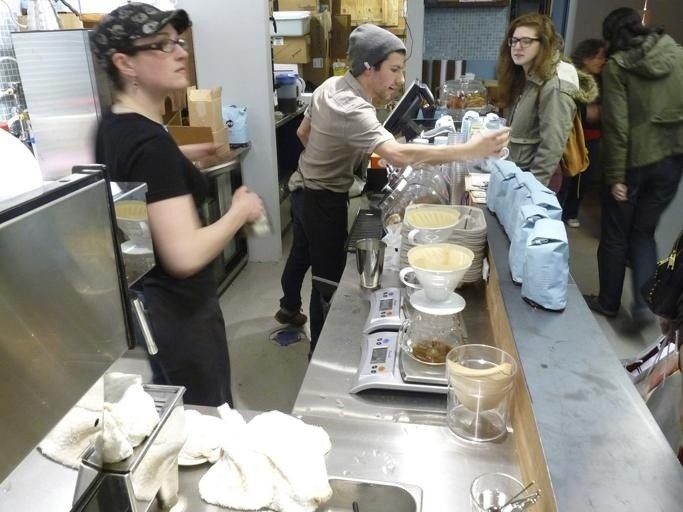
<box><xmin>317</xmin><ymin>474</ymin><xmax>424</xmax><ymax>512</ymax></box>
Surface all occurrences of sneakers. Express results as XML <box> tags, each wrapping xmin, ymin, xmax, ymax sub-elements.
<box><xmin>582</xmin><ymin>295</ymin><xmax>618</xmax><ymax>317</ymax></box>
<box><xmin>274</xmin><ymin>306</ymin><xmax>307</xmax><ymax>325</ymax></box>
<box><xmin>567</xmin><ymin>218</ymin><xmax>580</xmax><ymax>227</ymax></box>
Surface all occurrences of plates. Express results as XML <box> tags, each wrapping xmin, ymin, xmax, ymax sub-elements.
<box><xmin>407</xmin><ymin>292</ymin><xmax>468</xmax><ymax>316</ymax></box>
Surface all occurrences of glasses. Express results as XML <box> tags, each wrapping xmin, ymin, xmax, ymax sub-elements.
<box><xmin>123</xmin><ymin>38</ymin><xmax>187</xmax><ymax>53</ymax></box>
<box><xmin>507</xmin><ymin>36</ymin><xmax>541</xmax><ymax>48</ymax></box>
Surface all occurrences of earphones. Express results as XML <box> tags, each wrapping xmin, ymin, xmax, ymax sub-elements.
<box><xmin>363</xmin><ymin>62</ymin><xmax>371</xmax><ymax>71</ymax></box>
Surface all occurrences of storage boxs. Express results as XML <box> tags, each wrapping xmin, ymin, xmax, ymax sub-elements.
<box><xmin>273</xmin><ymin>9</ymin><xmax>313</xmax><ymax>107</ymax></box>
<box><xmin>164</xmin><ymin>85</ymin><xmax>232</xmax><ymax>173</ymax></box>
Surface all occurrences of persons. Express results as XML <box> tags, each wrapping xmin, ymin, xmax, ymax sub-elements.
<box><xmin>89</xmin><ymin>2</ymin><xmax>263</xmax><ymax>409</ymax></box>
<box><xmin>497</xmin><ymin>13</ymin><xmax>599</xmax><ymax>185</ymax></box>
<box><xmin>274</xmin><ymin>22</ymin><xmax>511</xmax><ymax>365</ymax></box>
<box><xmin>643</xmin><ymin>230</ymin><xmax>683</xmax><ymax>464</ymax></box>
<box><xmin>561</xmin><ymin>7</ymin><xmax>683</xmax><ymax>315</ymax></box>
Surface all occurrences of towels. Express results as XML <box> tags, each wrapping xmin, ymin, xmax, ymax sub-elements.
<box><xmin>36</xmin><ymin>370</ymin><xmax>335</xmax><ymax>512</ymax></box>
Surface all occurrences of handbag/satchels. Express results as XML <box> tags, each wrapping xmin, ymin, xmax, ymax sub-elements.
<box><xmin>641</xmin><ymin>229</ymin><xmax>683</xmax><ymax>321</ymax></box>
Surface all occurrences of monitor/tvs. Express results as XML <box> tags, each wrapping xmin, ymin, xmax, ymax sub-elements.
<box><xmin>383</xmin><ymin>79</ymin><xmax>434</xmax><ymax>136</ymax></box>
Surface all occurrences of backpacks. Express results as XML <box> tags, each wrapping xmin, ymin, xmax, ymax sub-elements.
<box><xmin>560</xmin><ymin>111</ymin><xmax>590</xmax><ymax>177</ymax></box>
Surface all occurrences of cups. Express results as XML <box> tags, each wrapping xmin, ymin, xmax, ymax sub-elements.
<box><xmin>444</xmin><ymin>343</ymin><xmax>520</xmax><ymax>445</ymax></box>
<box><xmin>399</xmin><ymin>207</ymin><xmax>475</xmax><ymax>301</ymax></box>
<box><xmin>400</xmin><ymin>311</ymin><xmax>468</xmax><ymax>365</ymax></box>
<box><xmin>447</xmin><ymin>133</ymin><xmax>469</xmax><ymax>204</ymax></box>
<box><xmin>470</xmin><ymin>473</ymin><xmax>533</xmax><ymax>512</ymax></box>
<box><xmin>353</xmin><ymin>238</ymin><xmax>387</xmax><ymax>289</ymax></box>
<box><xmin>274</xmin><ymin>73</ymin><xmax>302</xmax><ymax>112</ymax></box>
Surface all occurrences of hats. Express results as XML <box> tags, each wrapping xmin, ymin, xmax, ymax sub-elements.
<box><xmin>91</xmin><ymin>2</ymin><xmax>192</xmax><ymax>60</ymax></box>
<box><xmin>349</xmin><ymin>24</ymin><xmax>407</xmax><ymax>79</ymax></box>
